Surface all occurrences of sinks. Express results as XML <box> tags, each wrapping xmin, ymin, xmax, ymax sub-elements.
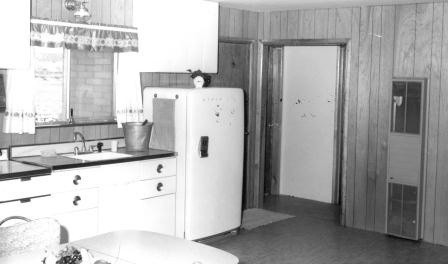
<box><xmin>61</xmin><ymin>150</ymin><xmax>133</xmax><ymax>162</ymax></box>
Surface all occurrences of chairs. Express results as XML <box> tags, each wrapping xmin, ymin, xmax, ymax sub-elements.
<box><xmin>0</xmin><ymin>216</ymin><xmax>61</xmax><ymax>256</ymax></box>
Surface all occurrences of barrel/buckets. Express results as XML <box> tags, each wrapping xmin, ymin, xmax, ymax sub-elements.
<box><xmin>120</xmin><ymin>120</ymin><xmax>154</xmax><ymax>152</ymax></box>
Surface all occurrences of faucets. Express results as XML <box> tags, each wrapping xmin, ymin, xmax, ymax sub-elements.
<box><xmin>73</xmin><ymin>131</ymin><xmax>87</xmax><ymax>152</ymax></box>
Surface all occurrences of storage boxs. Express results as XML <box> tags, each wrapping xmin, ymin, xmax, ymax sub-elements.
<box><xmin>54</xmin><ymin>166</ymin><xmax>98</xmax><ymax>243</ymax></box>
<box><xmin>96</xmin><ymin>158</ymin><xmax>176</xmax><ymax>238</ymax></box>
<box><xmin>0</xmin><ymin>172</ymin><xmax>52</xmax><ymax>225</ymax></box>
<box><xmin>123</xmin><ymin>122</ymin><xmax>154</xmax><ymax>153</ymax></box>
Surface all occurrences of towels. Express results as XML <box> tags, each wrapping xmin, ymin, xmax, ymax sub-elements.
<box><xmin>4</xmin><ymin>67</ymin><xmax>35</xmax><ymax>134</ymax></box>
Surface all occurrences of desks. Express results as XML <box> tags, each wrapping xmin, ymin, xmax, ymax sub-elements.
<box><xmin>0</xmin><ymin>230</ymin><xmax>241</xmax><ymax>264</ymax></box>
